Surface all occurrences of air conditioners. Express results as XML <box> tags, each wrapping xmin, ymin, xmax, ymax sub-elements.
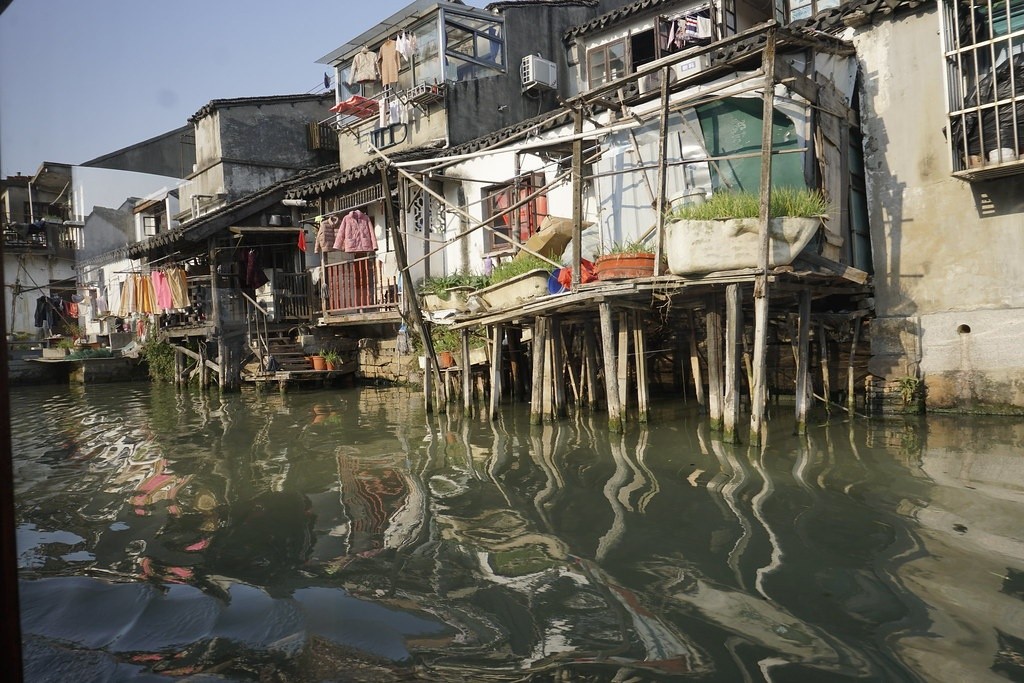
<box><xmin>637</xmin><ymin>46</ymin><xmax>709</xmax><ymax>99</ymax></box>
<box><xmin>521</xmin><ymin>56</ymin><xmax>559</xmax><ymax>91</ymax></box>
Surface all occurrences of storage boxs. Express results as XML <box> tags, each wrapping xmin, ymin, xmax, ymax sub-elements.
<box><xmin>511</xmin><ymin>215</ymin><xmax>595</xmax><ymax>261</ymax></box>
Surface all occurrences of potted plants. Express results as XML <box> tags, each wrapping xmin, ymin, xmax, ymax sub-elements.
<box><xmin>416</xmin><ymin>269</ymin><xmax>484</xmax><ymax>314</ymax></box>
<box><xmin>312</xmin><ymin>403</ymin><xmax>343</xmax><ymax>426</ymax></box>
<box><xmin>303</xmin><ymin>347</ymin><xmax>343</xmax><ymax>370</ymax></box>
<box><xmin>661</xmin><ymin>185</ymin><xmax>841</xmax><ymax>276</ymax></box>
<box><xmin>594</xmin><ymin>233</ymin><xmax>667</xmax><ymax>280</ymax></box>
<box><xmin>42</xmin><ymin>338</ymin><xmax>93</xmax><ymax>360</ymax></box>
<box><xmin>467</xmin><ymin>244</ymin><xmax>567</xmax><ymax>313</ymax></box>
<box><xmin>412</xmin><ymin>323</ymin><xmax>490</xmax><ymax>370</ymax></box>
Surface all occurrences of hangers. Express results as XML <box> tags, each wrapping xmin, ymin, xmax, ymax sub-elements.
<box><xmin>361</xmin><ymin>24</ymin><xmax>417</xmax><ymax>50</ymax></box>
<box><xmin>328</xmin><ymin>207</ymin><xmax>358</xmax><ymax>220</ymax></box>
<box><xmin>125</xmin><ymin>260</ymin><xmax>183</xmax><ymax>280</ymax></box>
